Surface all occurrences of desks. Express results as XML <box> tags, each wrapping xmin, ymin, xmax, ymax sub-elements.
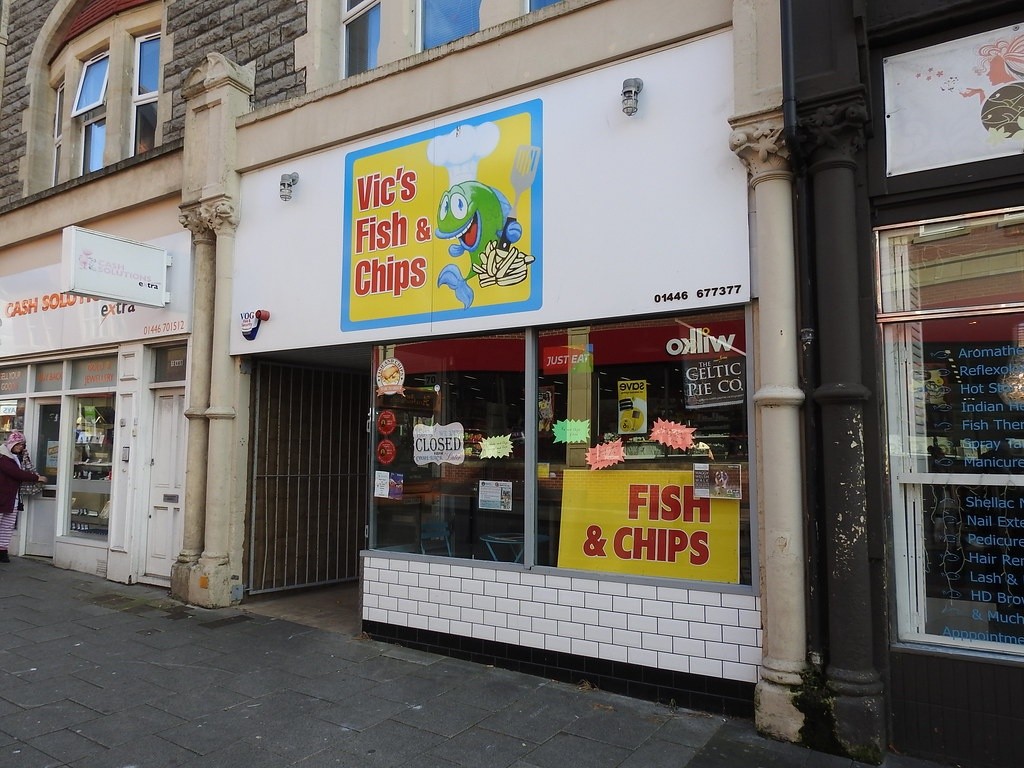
<box><xmin>480</xmin><ymin>533</ymin><xmax>549</xmax><ymax>562</ymax></box>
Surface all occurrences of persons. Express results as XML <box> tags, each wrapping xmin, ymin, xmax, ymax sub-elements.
<box><xmin>0</xmin><ymin>429</ymin><xmax>47</xmax><ymax>563</ymax></box>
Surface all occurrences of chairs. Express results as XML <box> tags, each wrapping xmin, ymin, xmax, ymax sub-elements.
<box><xmin>420</xmin><ymin>522</ymin><xmax>451</xmax><ymax>556</ymax></box>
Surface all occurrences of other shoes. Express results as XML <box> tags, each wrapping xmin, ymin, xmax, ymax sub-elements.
<box><xmin>0</xmin><ymin>550</ymin><xmax>10</xmax><ymax>563</ymax></box>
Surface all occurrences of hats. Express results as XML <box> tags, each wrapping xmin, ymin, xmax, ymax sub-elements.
<box><xmin>7</xmin><ymin>428</ymin><xmax>27</xmax><ymax>451</ymax></box>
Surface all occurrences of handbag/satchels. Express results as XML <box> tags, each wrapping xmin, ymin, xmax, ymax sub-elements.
<box><xmin>21</xmin><ymin>450</ymin><xmax>42</xmax><ymax>496</ymax></box>
